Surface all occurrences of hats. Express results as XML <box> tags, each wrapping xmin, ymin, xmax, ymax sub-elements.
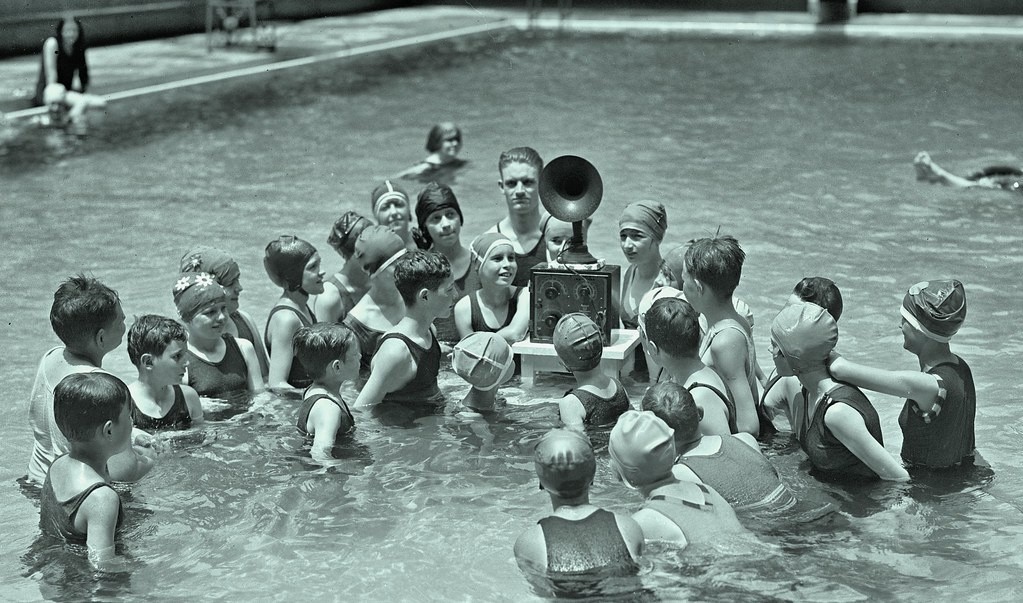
<box><xmin>449</xmin><ymin>330</ymin><xmax>513</xmax><ymax>391</ymax></box>
<box><xmin>699</xmin><ymin>296</ymin><xmax>754</xmax><ymax>340</ymax></box>
<box><xmin>175</xmin><ymin>273</ymin><xmax>223</xmax><ymax>322</ymax></box>
<box><xmin>371</xmin><ymin>180</ymin><xmax>408</xmax><ymax>218</ymax></box>
<box><xmin>610</xmin><ymin>409</ymin><xmax>676</xmax><ymax>489</ymax></box>
<box><xmin>769</xmin><ymin>300</ymin><xmax>839</xmax><ymax>374</ymax></box>
<box><xmin>664</xmin><ymin>243</ymin><xmax>690</xmax><ymax>293</ymax></box>
<box><xmin>900</xmin><ymin>276</ymin><xmax>966</xmax><ymax>341</ymax></box>
<box><xmin>416</xmin><ymin>181</ymin><xmax>464</xmax><ymax>233</ymax></box>
<box><xmin>471</xmin><ymin>231</ymin><xmax>515</xmax><ymax>280</ymax></box>
<box><xmin>552</xmin><ymin>311</ymin><xmax>602</xmax><ymax>370</ymax></box>
<box><xmin>352</xmin><ymin>224</ymin><xmax>406</xmax><ymax>279</ymax></box>
<box><xmin>325</xmin><ymin>210</ymin><xmax>373</xmax><ymax>261</ymax></box>
<box><xmin>538</xmin><ymin>210</ymin><xmax>593</xmax><ymax>241</ymax></box>
<box><xmin>180</xmin><ymin>245</ymin><xmax>240</xmax><ymax>288</ymax></box>
<box><xmin>262</xmin><ymin>235</ymin><xmax>316</xmax><ymax>294</ymax></box>
<box><xmin>426</xmin><ymin>121</ymin><xmax>463</xmax><ymax>152</ymax></box>
<box><xmin>618</xmin><ymin>199</ymin><xmax>669</xmax><ymax>244</ymax></box>
<box><xmin>534</xmin><ymin>428</ymin><xmax>596</xmax><ymax>497</ymax></box>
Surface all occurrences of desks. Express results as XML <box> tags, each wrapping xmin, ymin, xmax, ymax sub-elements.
<box><xmin>513</xmin><ymin>330</ymin><xmax>640</xmax><ymax>383</ymax></box>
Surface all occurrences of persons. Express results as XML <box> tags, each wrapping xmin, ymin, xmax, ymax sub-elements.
<box><xmin>639</xmin><ymin>381</ymin><xmax>819</xmax><ymax>513</ymax></box>
<box><xmin>453</xmin><ymin>231</ymin><xmax>530</xmax><ymax>375</ymax></box>
<box><xmin>41</xmin><ymin>372</ymin><xmax>134</xmax><ymax>563</ymax></box>
<box><xmin>638</xmin><ymin>225</ymin><xmax>760</xmax><ymax>438</ymax></box>
<box><xmin>396</xmin><ymin>122</ymin><xmax>462</xmax><ymax>178</ymax></box>
<box><xmin>468</xmin><ymin>148</ymin><xmax>546</xmax><ymax>287</ymax></box>
<box><xmin>827</xmin><ymin>280</ymin><xmax>976</xmax><ymax>468</ymax></box>
<box><xmin>514</xmin><ymin>428</ymin><xmax>644</xmax><ymax>571</ymax></box>
<box><xmin>263</xmin><ymin>178</ymin><xmax>481</xmax><ymax>457</ymax></box>
<box><xmin>527</xmin><ymin>211</ymin><xmax>592</xmax><ymax>286</ymax></box>
<box><xmin>619</xmin><ymin>200</ymin><xmax>668</xmax><ymax>358</ymax></box>
<box><xmin>608</xmin><ymin>410</ymin><xmax>743</xmax><ymax>542</ymax></box>
<box><xmin>913</xmin><ymin>149</ymin><xmax>974</xmax><ymax>188</ymax></box>
<box><xmin>30</xmin><ymin>12</ymin><xmax>107</xmax><ymax>119</ymax></box>
<box><xmin>553</xmin><ymin>312</ymin><xmax>630</xmax><ymax>424</ymax></box>
<box><xmin>452</xmin><ymin>331</ymin><xmax>515</xmax><ymax>407</ymax></box>
<box><xmin>174</xmin><ymin>246</ymin><xmax>270</xmax><ymax>396</ymax></box>
<box><xmin>27</xmin><ymin>270</ymin><xmax>157</xmax><ymax>488</ymax></box>
<box><xmin>126</xmin><ymin>313</ymin><xmax>203</xmax><ymax>433</ymax></box>
<box><xmin>760</xmin><ymin>278</ymin><xmax>909</xmax><ymax>482</ymax></box>
